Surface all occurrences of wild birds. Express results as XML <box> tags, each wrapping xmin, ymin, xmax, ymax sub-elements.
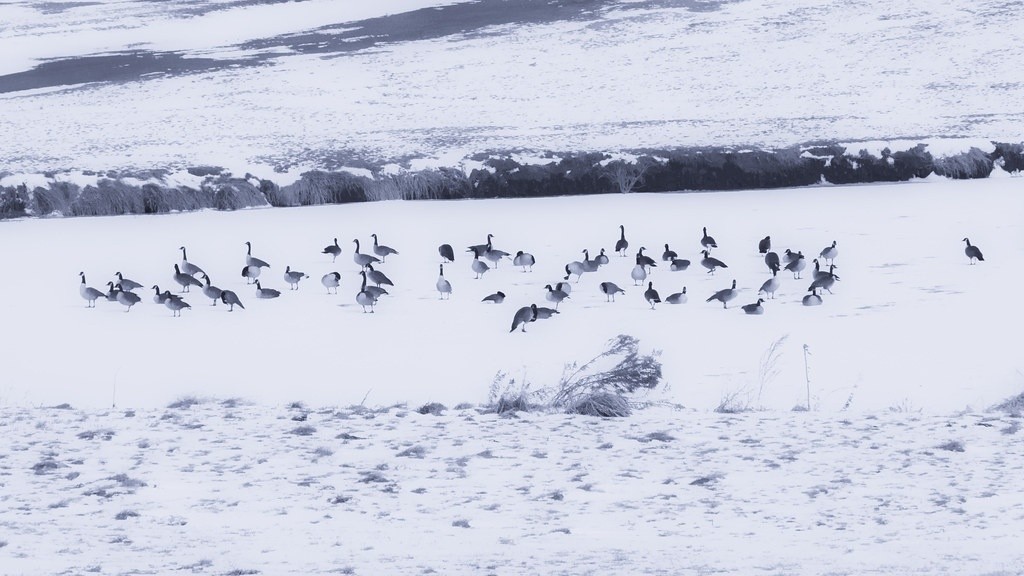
<box><xmin>252</xmin><ymin>279</ymin><xmax>281</xmax><ymax>299</ymax></box>
<box><xmin>439</xmin><ymin>244</ymin><xmax>455</xmax><ymax>265</ymax></box>
<box><xmin>105</xmin><ymin>271</ymin><xmax>144</xmax><ymax>312</ymax></box>
<box><xmin>321</xmin><ymin>272</ymin><xmax>340</xmax><ymax>295</ymax></box>
<box><xmin>508</xmin><ymin>304</ymin><xmax>559</xmax><ymax>333</ymax></box>
<box><xmin>179</xmin><ymin>246</ymin><xmax>206</xmax><ymax>280</ymax></box>
<box><xmin>284</xmin><ymin>265</ymin><xmax>310</xmax><ymax>291</ymax></box>
<box><xmin>321</xmin><ymin>234</ymin><xmax>400</xmax><ymax>315</ymax></box>
<box><xmin>962</xmin><ymin>237</ymin><xmax>985</xmax><ymax>265</ymax></box>
<box><xmin>162</xmin><ymin>290</ymin><xmax>191</xmax><ymax>317</ymax></box>
<box><xmin>201</xmin><ymin>275</ymin><xmax>222</xmax><ymax>306</ymax></box>
<box><xmin>79</xmin><ymin>271</ymin><xmax>109</xmax><ymax>308</ymax></box>
<box><xmin>219</xmin><ymin>290</ymin><xmax>245</xmax><ymax>312</ymax></box>
<box><xmin>466</xmin><ymin>232</ymin><xmax>511</xmax><ymax>280</ymax></box>
<box><xmin>149</xmin><ymin>284</ymin><xmax>184</xmax><ymax>303</ymax></box>
<box><xmin>245</xmin><ymin>241</ymin><xmax>271</xmax><ymax>268</ymax></box>
<box><xmin>480</xmin><ymin>290</ymin><xmax>505</xmax><ymax>305</ymax></box>
<box><xmin>174</xmin><ymin>263</ymin><xmax>204</xmax><ymax>294</ymax></box>
<box><xmin>512</xmin><ymin>226</ymin><xmax>842</xmax><ymax>316</ymax></box>
<box><xmin>242</xmin><ymin>266</ymin><xmax>261</xmax><ymax>284</ymax></box>
<box><xmin>436</xmin><ymin>263</ymin><xmax>454</xmax><ymax>300</ymax></box>
